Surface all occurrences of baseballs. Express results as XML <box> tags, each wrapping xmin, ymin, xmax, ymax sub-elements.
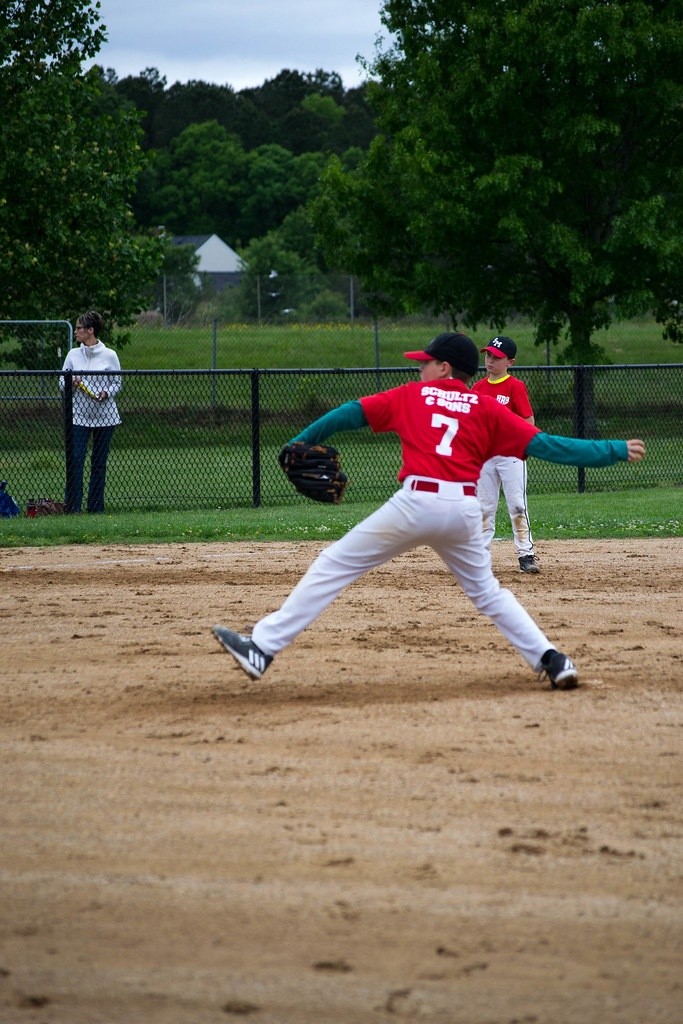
<box><xmin>633</xmin><ymin>452</ymin><xmax>643</xmax><ymax>462</ymax></box>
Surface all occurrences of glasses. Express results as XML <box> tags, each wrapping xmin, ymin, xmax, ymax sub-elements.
<box><xmin>74</xmin><ymin>327</ymin><xmax>83</xmax><ymax>331</ymax></box>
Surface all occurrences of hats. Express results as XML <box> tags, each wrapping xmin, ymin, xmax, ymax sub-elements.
<box><xmin>480</xmin><ymin>335</ymin><xmax>516</xmax><ymax>358</ymax></box>
<box><xmin>403</xmin><ymin>331</ymin><xmax>479</xmax><ymax>376</ymax></box>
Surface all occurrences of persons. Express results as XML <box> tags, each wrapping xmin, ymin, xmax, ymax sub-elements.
<box><xmin>60</xmin><ymin>312</ymin><xmax>122</xmax><ymax>514</ymax></box>
<box><xmin>472</xmin><ymin>336</ymin><xmax>540</xmax><ymax>572</ymax></box>
<box><xmin>212</xmin><ymin>333</ymin><xmax>644</xmax><ymax>691</ymax></box>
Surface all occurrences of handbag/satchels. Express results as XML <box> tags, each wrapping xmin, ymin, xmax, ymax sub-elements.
<box><xmin>0</xmin><ymin>481</ymin><xmax>20</xmax><ymax>518</ymax></box>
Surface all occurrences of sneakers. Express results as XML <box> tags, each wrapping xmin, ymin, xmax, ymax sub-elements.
<box><xmin>537</xmin><ymin>652</ymin><xmax>578</xmax><ymax>689</ymax></box>
<box><xmin>209</xmin><ymin>624</ymin><xmax>274</xmax><ymax>681</ymax></box>
<box><xmin>517</xmin><ymin>555</ymin><xmax>540</xmax><ymax>574</ymax></box>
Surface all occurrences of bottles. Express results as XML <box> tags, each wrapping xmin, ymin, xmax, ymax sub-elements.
<box><xmin>78</xmin><ymin>376</ymin><xmax>100</xmax><ymax>400</ymax></box>
<box><xmin>26</xmin><ymin>498</ymin><xmax>36</xmax><ymax>517</ymax></box>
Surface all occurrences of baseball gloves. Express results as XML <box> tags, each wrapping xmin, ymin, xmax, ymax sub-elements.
<box><xmin>278</xmin><ymin>439</ymin><xmax>349</xmax><ymax>507</ymax></box>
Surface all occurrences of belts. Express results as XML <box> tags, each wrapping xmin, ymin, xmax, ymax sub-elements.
<box><xmin>410</xmin><ymin>480</ymin><xmax>478</xmax><ymax>496</ymax></box>
